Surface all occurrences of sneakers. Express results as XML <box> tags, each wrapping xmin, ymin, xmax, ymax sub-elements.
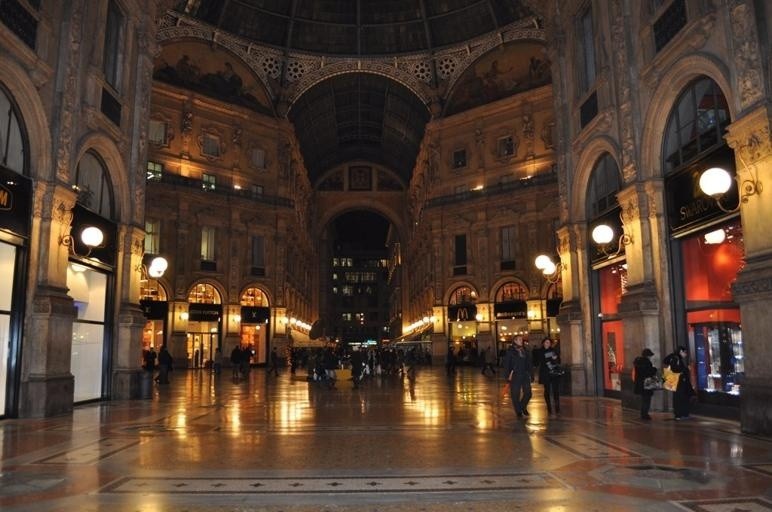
<box><xmin>675</xmin><ymin>415</ymin><xmax>696</xmax><ymax>420</ymax></box>
<box><xmin>641</xmin><ymin>414</ymin><xmax>652</xmax><ymax>421</ymax></box>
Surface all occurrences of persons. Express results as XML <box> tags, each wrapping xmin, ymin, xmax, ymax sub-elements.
<box><xmin>633</xmin><ymin>349</ymin><xmax>657</xmax><ymax>420</ymax></box>
<box><xmin>291</xmin><ymin>344</ymin><xmax>433</xmax><ymax>390</ymax></box>
<box><xmin>664</xmin><ymin>346</ymin><xmax>694</xmax><ymax>421</ymax></box>
<box><xmin>143</xmin><ymin>344</ymin><xmax>172</xmax><ymax>385</ymax></box>
<box><xmin>267</xmin><ymin>346</ymin><xmax>281</xmax><ymax>376</ymax></box>
<box><xmin>504</xmin><ymin>335</ymin><xmax>562</xmax><ymax>416</ymax></box>
<box><xmin>213</xmin><ymin>345</ymin><xmax>253</xmax><ymax>376</ymax></box>
<box><xmin>447</xmin><ymin>344</ymin><xmax>504</xmax><ymax>376</ymax></box>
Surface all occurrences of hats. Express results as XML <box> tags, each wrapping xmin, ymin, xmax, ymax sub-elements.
<box><xmin>642</xmin><ymin>349</ymin><xmax>654</xmax><ymax>356</ymax></box>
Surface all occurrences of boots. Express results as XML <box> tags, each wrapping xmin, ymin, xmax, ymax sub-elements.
<box><xmin>556</xmin><ymin>408</ymin><xmax>560</xmax><ymax>417</ymax></box>
<box><xmin>548</xmin><ymin>408</ymin><xmax>553</xmax><ymax>415</ymax></box>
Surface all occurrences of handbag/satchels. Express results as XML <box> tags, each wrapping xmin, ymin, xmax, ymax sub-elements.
<box><xmin>661</xmin><ymin>364</ymin><xmax>680</xmax><ymax>392</ymax></box>
<box><xmin>644</xmin><ymin>375</ymin><xmax>662</xmax><ymax>390</ymax></box>
<box><xmin>552</xmin><ymin>365</ymin><xmax>566</xmax><ymax>377</ymax></box>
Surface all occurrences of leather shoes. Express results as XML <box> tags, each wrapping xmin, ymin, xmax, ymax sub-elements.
<box><xmin>516</xmin><ymin>409</ymin><xmax>529</xmax><ymax>418</ymax></box>
